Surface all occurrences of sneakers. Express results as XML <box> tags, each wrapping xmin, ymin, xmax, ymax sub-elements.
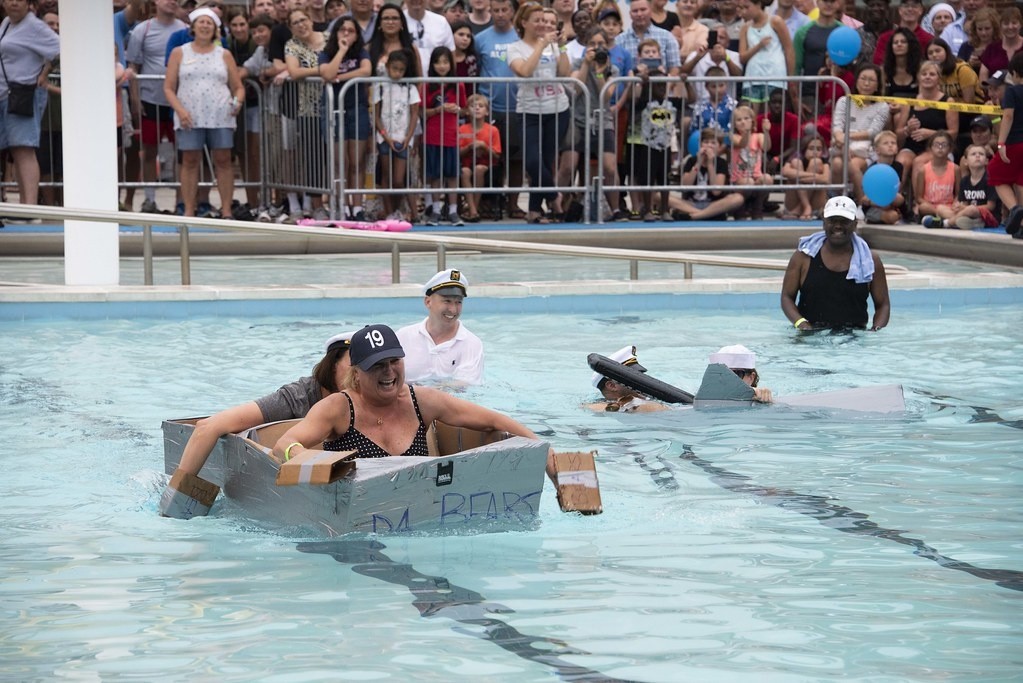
<box><xmin>955</xmin><ymin>216</ymin><xmax>985</xmax><ymax>229</ymax></box>
<box><xmin>921</xmin><ymin>215</ymin><xmax>943</xmax><ymax>229</ymax></box>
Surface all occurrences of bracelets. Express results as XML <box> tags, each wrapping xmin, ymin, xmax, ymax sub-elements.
<box><xmin>596</xmin><ymin>74</ymin><xmax>604</xmax><ymax>78</ymax></box>
<box><xmin>997</xmin><ymin>142</ymin><xmax>1004</xmax><ymax>148</ymax></box>
<box><xmin>336</xmin><ymin>76</ymin><xmax>340</xmax><ymax>83</ymax></box>
<box><xmin>794</xmin><ymin>317</ymin><xmax>808</xmax><ymax>328</ymax></box>
<box><xmin>872</xmin><ymin>326</ymin><xmax>880</xmax><ymax>331</ymax></box>
<box><xmin>285</xmin><ymin>442</ymin><xmax>304</xmax><ymax>461</ymax></box>
<box><xmin>726</xmin><ymin>55</ymin><xmax>730</xmax><ymax>62</ymax></box>
<box><xmin>380</xmin><ymin>130</ymin><xmax>387</xmax><ymax>135</ymax></box>
<box><xmin>560</xmin><ymin>46</ymin><xmax>567</xmax><ymax>54</ymax></box>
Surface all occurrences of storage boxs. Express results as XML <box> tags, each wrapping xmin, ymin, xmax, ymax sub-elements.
<box><xmin>163</xmin><ymin>415</ymin><xmax>553</xmax><ymax>536</ymax></box>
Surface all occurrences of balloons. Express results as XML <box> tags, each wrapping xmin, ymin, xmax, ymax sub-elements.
<box><xmin>862</xmin><ymin>163</ymin><xmax>899</xmax><ymax>208</ymax></box>
<box><xmin>689</xmin><ymin>128</ymin><xmax>700</xmax><ymax>155</ymax></box>
<box><xmin>827</xmin><ymin>26</ymin><xmax>862</xmax><ymax>66</ymax></box>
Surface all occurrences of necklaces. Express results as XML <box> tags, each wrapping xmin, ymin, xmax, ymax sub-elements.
<box><xmin>357</xmin><ymin>394</ymin><xmax>399</xmax><ymax>425</ymax></box>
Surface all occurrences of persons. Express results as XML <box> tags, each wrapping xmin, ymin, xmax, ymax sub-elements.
<box><xmin>1</xmin><ymin>0</ymin><xmax>1023</xmax><ymax>243</ymax></box>
<box><xmin>273</xmin><ymin>323</ymin><xmax>602</xmax><ymax>514</ymax></box>
<box><xmin>157</xmin><ymin>330</ymin><xmax>360</xmax><ymax>515</ymax></box>
<box><xmin>584</xmin><ymin>344</ymin><xmax>773</xmax><ymax>411</ymax></box>
<box><xmin>393</xmin><ymin>267</ymin><xmax>484</xmax><ymax>384</ymax></box>
<box><xmin>781</xmin><ymin>196</ymin><xmax>891</xmax><ymax>332</ymax></box>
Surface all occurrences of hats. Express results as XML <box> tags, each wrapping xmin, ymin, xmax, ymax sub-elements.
<box><xmin>709</xmin><ymin>344</ymin><xmax>756</xmax><ymax>372</ymax></box>
<box><xmin>982</xmin><ymin>70</ymin><xmax>1015</xmax><ymax>87</ymax></box>
<box><xmin>324</xmin><ymin>0</ymin><xmax>346</xmax><ymax>14</ymax></box>
<box><xmin>423</xmin><ymin>268</ymin><xmax>468</xmax><ymax>299</ymax></box>
<box><xmin>969</xmin><ymin>114</ymin><xmax>992</xmax><ymax>129</ymax></box>
<box><xmin>592</xmin><ymin>344</ymin><xmax>646</xmax><ymax>393</ymax></box>
<box><xmin>326</xmin><ymin>331</ymin><xmax>358</xmax><ymax>350</ymax></box>
<box><xmin>188</xmin><ymin>8</ymin><xmax>222</xmax><ymax>27</ymax></box>
<box><xmin>822</xmin><ymin>195</ymin><xmax>857</xmax><ymax>221</ymax></box>
<box><xmin>350</xmin><ymin>323</ymin><xmax>405</xmax><ymax>372</ymax></box>
<box><xmin>597</xmin><ymin>7</ymin><xmax>620</xmax><ymax>22</ymax></box>
<box><xmin>442</xmin><ymin>0</ymin><xmax>466</xmax><ymax>14</ymax></box>
<box><xmin>929</xmin><ymin>3</ymin><xmax>956</xmax><ymax>26</ymax></box>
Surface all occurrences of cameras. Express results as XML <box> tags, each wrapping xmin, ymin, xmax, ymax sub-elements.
<box><xmin>593</xmin><ymin>45</ymin><xmax>609</xmax><ymax>64</ymax></box>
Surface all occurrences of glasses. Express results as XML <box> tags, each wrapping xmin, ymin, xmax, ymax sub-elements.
<box><xmin>339</xmin><ymin>27</ymin><xmax>355</xmax><ymax>34</ymax></box>
<box><xmin>381</xmin><ymin>16</ymin><xmax>401</xmax><ymax>23</ymax></box>
<box><xmin>521</xmin><ymin>2</ymin><xmax>539</xmax><ymax>18</ymax></box>
<box><xmin>290</xmin><ymin>16</ymin><xmax>310</xmax><ymax>28</ymax></box>
<box><xmin>733</xmin><ymin>369</ymin><xmax>751</xmax><ymax>379</ymax></box>
<box><xmin>931</xmin><ymin>142</ymin><xmax>949</xmax><ymax>148</ymax></box>
<box><xmin>899</xmin><ymin>3</ymin><xmax>921</xmax><ymax>9</ymax></box>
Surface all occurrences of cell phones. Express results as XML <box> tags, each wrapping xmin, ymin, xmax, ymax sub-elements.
<box><xmin>555</xmin><ymin>21</ymin><xmax>564</xmax><ymax>36</ymax></box>
<box><xmin>708</xmin><ymin>30</ymin><xmax>717</xmax><ymax>50</ymax></box>
<box><xmin>640</xmin><ymin>59</ymin><xmax>661</xmax><ymax>71</ymax></box>
<box><xmin>233</xmin><ymin>97</ymin><xmax>237</xmax><ymax>111</ymax></box>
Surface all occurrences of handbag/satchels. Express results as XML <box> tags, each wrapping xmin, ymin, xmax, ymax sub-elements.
<box><xmin>7</xmin><ymin>82</ymin><xmax>36</xmax><ymax>116</ymax></box>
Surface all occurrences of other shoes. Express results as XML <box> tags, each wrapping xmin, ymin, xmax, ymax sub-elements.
<box><xmin>817</xmin><ymin>201</ymin><xmax>818</xmax><ymax>202</ymax></box>
<box><xmin>6</xmin><ymin>203</ymin><xmax>818</xmax><ymax>226</ymax></box>
<box><xmin>1006</xmin><ymin>207</ymin><xmax>1023</xmax><ymax>238</ymax></box>
